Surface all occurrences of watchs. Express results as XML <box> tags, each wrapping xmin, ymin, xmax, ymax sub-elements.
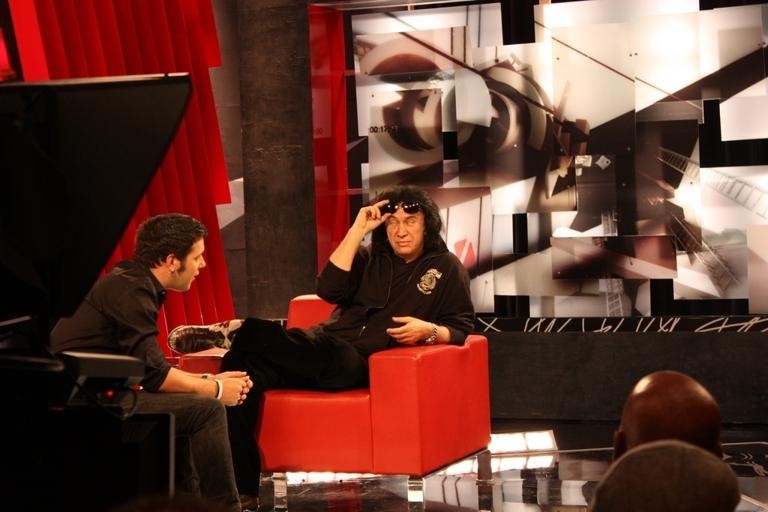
<box><xmin>425</xmin><ymin>321</ymin><xmax>440</xmax><ymax>345</ymax></box>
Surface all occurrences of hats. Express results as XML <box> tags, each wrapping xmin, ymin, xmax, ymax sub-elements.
<box><xmin>589</xmin><ymin>440</ymin><xmax>740</xmax><ymax>511</ymax></box>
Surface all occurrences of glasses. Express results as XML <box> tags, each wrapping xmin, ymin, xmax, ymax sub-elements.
<box><xmin>380</xmin><ymin>201</ymin><xmax>419</xmax><ymax>215</ymax></box>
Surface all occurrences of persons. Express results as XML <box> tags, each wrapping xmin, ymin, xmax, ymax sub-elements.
<box><xmin>221</xmin><ymin>186</ymin><xmax>475</xmax><ymax>512</ymax></box>
<box><xmin>610</xmin><ymin>371</ymin><xmax>768</xmax><ymax>511</ymax></box>
<box><xmin>42</xmin><ymin>214</ymin><xmax>254</xmax><ymax>512</ymax></box>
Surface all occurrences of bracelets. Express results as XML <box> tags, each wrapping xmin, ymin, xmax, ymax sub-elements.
<box><xmin>202</xmin><ymin>373</ymin><xmax>209</xmax><ymax>378</ymax></box>
<box><xmin>215</xmin><ymin>379</ymin><xmax>223</xmax><ymax>399</ymax></box>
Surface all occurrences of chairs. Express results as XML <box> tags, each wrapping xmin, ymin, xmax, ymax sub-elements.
<box><xmin>2</xmin><ymin>331</ymin><xmax>175</xmax><ymax>510</ymax></box>
<box><xmin>177</xmin><ymin>293</ymin><xmax>491</xmax><ymax>500</ymax></box>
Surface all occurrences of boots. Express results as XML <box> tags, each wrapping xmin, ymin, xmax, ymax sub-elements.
<box><xmin>168</xmin><ymin>319</ymin><xmax>245</xmax><ymax>353</ymax></box>
<box><xmin>239</xmin><ymin>495</ymin><xmax>258</xmax><ymax>511</ymax></box>
